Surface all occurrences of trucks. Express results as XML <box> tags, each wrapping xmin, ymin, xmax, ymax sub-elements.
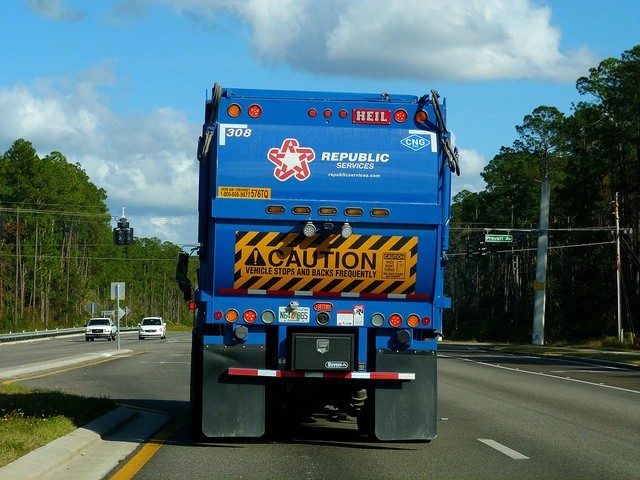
<box><xmin>175</xmin><ymin>82</ymin><xmax>459</xmax><ymax>444</ymax></box>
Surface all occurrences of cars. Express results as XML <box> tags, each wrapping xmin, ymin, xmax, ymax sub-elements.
<box><xmin>137</xmin><ymin>316</ymin><xmax>167</xmax><ymax>340</ymax></box>
<box><xmin>85</xmin><ymin>317</ymin><xmax>117</xmax><ymax>342</ymax></box>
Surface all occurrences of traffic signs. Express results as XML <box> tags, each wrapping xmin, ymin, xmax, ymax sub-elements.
<box><xmin>485</xmin><ymin>235</ymin><xmax>513</xmax><ymax>243</ymax></box>
